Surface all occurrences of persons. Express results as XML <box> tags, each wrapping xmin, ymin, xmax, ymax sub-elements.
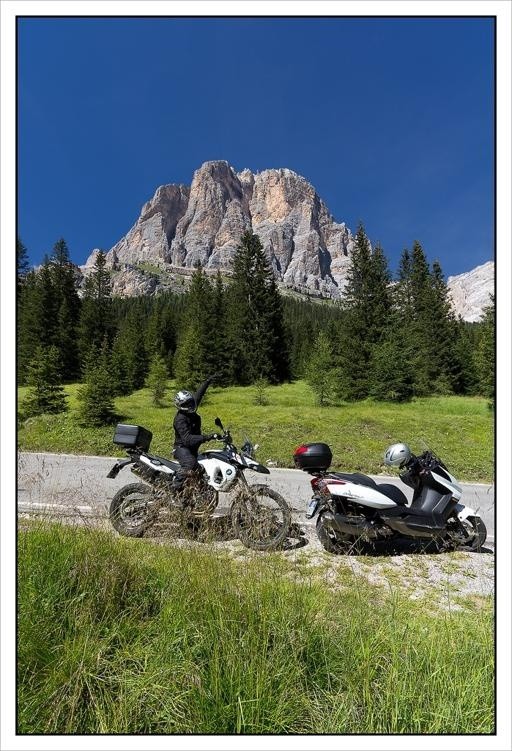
<box><xmin>172</xmin><ymin>371</ymin><xmax>222</xmax><ymax>534</ymax></box>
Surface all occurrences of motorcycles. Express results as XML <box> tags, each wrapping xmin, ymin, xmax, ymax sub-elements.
<box><xmin>105</xmin><ymin>416</ymin><xmax>291</xmax><ymax>550</ymax></box>
<box><xmin>293</xmin><ymin>437</ymin><xmax>487</xmax><ymax>552</ymax></box>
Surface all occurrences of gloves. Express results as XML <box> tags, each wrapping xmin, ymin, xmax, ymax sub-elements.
<box><xmin>206</xmin><ymin>433</ymin><xmax>222</xmax><ymax>442</ymax></box>
<box><xmin>207</xmin><ymin>374</ymin><xmax>223</xmax><ymax>382</ymax></box>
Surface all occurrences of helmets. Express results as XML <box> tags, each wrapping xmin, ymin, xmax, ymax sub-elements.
<box><xmin>383</xmin><ymin>442</ymin><xmax>412</xmax><ymax>468</ymax></box>
<box><xmin>173</xmin><ymin>390</ymin><xmax>197</xmax><ymax>414</ymax></box>
